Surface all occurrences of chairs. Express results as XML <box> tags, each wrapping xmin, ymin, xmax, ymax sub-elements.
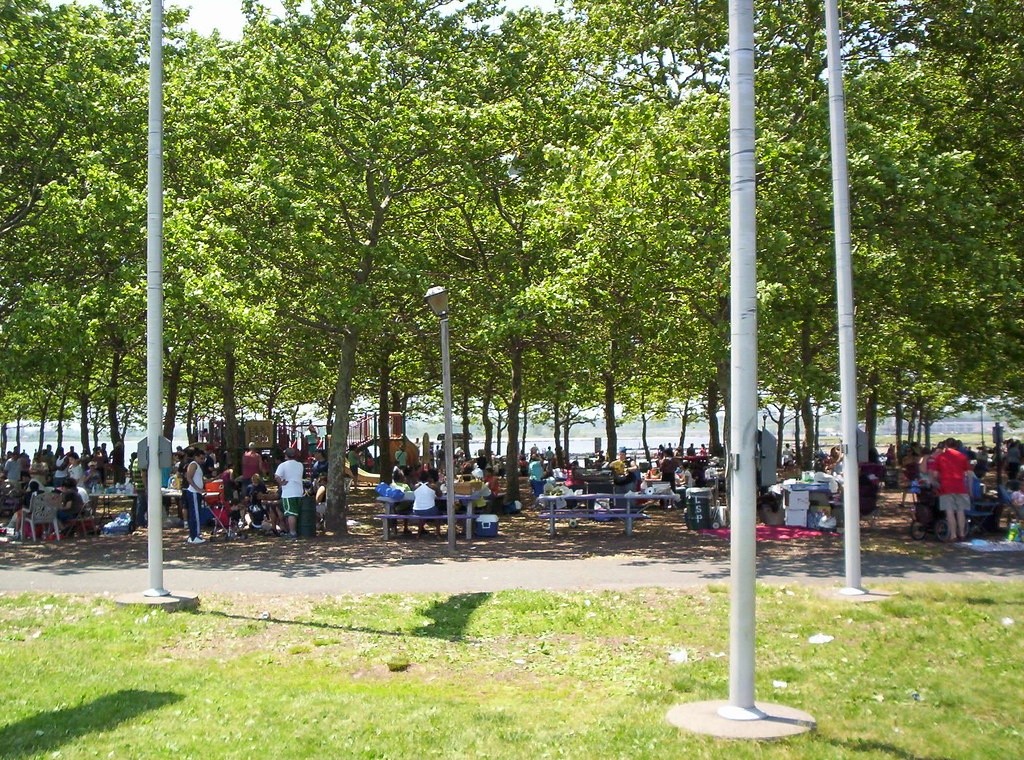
<box><xmin>21</xmin><ymin>493</ymin><xmax>63</xmax><ymax>543</ymax></box>
<box><xmin>61</xmin><ymin>493</ymin><xmax>121</xmax><ymax>540</ymax></box>
<box><xmin>964</xmin><ymin>498</ymin><xmax>999</xmax><ymax>534</ymax></box>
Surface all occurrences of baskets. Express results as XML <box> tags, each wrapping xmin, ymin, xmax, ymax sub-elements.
<box><xmin>454</xmin><ymin>474</ymin><xmax>482</xmax><ymax>495</ymax></box>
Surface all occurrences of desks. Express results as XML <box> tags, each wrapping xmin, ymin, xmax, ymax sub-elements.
<box><xmin>540</xmin><ymin>494</ymin><xmax>679</xmax><ymax>538</ymax></box>
<box><xmin>584</xmin><ymin>481</ymin><xmax>617</xmax><ymax>510</ymax></box>
<box><xmin>376</xmin><ymin>493</ymin><xmax>482</xmax><ymax>540</ymax></box>
<box><xmin>89</xmin><ymin>489</ymin><xmax>219</xmax><ymax>527</ymax></box>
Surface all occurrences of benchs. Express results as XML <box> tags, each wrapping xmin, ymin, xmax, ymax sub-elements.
<box><xmin>478</xmin><ymin>493</ymin><xmax>509</xmax><ymax>517</ymax></box>
<box><xmin>541</xmin><ymin>509</ymin><xmax>639</xmax><ymax>514</ymax></box>
<box><xmin>374</xmin><ymin>513</ymin><xmax>478</xmax><ymax>520</ymax></box>
<box><xmin>539</xmin><ymin>513</ymin><xmax>647</xmax><ymax>519</ymax></box>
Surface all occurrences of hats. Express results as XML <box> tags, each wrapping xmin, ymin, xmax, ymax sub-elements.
<box><xmin>399</xmin><ymin>444</ymin><xmax>406</xmax><ymax>450</ymax></box>
<box><xmin>284</xmin><ymin>448</ymin><xmax>295</xmax><ymax>457</ymax></box>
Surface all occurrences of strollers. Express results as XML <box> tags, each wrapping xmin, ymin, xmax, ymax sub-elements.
<box><xmin>203</xmin><ymin>491</ymin><xmax>248</xmax><ymax>545</ymax></box>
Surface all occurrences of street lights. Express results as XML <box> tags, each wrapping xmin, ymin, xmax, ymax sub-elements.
<box><xmin>425</xmin><ymin>284</ymin><xmax>460</xmax><ymax>548</ymax></box>
<box><xmin>763</xmin><ymin>414</ymin><xmax>768</xmax><ymax>428</ymax></box>
<box><xmin>980</xmin><ymin>403</ymin><xmax>987</xmax><ymax>446</ymax></box>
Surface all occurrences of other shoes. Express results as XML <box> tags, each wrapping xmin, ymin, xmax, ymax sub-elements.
<box><xmin>943</xmin><ymin>535</ymin><xmax>958</xmax><ymax>542</ymax></box>
<box><xmin>898</xmin><ymin>503</ymin><xmax>905</xmax><ymax>508</ymax></box>
<box><xmin>187</xmin><ymin>536</ymin><xmax>206</xmax><ymax>544</ymax></box>
<box><xmin>279</xmin><ymin>531</ymin><xmax>287</xmax><ymax>536</ymax></box>
<box><xmin>958</xmin><ymin>535</ymin><xmax>967</xmax><ymax>542</ymax></box>
<box><xmin>269</xmin><ymin>528</ymin><xmax>277</xmax><ymax>534</ymax></box>
<box><xmin>284</xmin><ymin>531</ymin><xmax>298</xmax><ymax>539</ymax></box>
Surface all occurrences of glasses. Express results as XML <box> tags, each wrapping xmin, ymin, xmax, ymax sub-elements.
<box><xmin>906</xmin><ymin>450</ymin><xmax>911</xmax><ymax>452</ymax></box>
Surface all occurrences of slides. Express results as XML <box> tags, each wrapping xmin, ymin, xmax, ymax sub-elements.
<box><xmin>344</xmin><ymin>458</ymin><xmax>380</xmax><ymax>483</ymax></box>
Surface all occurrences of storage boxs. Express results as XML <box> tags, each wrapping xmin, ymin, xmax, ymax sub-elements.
<box><xmin>711</xmin><ymin>506</ymin><xmax>727</xmax><ymax>525</ymax></box>
<box><xmin>77</xmin><ymin>519</ymin><xmax>96</xmax><ymax>532</ymax></box>
<box><xmin>476</xmin><ymin>514</ymin><xmax>500</xmax><ymax>536</ymax></box>
<box><xmin>783</xmin><ymin>484</ymin><xmax>845</xmax><ymax>530</ymax></box>
<box><xmin>205</xmin><ymin>478</ymin><xmax>232</xmax><ymax>527</ymax></box>
<box><xmin>26</xmin><ymin>521</ymin><xmax>44</xmax><ymax>539</ymax></box>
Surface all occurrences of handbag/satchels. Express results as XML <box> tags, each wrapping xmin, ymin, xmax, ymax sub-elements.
<box><xmin>910</xmin><ymin>479</ymin><xmax>920</xmax><ymax>493</ymax></box>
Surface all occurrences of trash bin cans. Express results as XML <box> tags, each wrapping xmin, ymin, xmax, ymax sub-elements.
<box><xmin>686</xmin><ymin>487</ymin><xmax>710</xmax><ymax>529</ymax></box>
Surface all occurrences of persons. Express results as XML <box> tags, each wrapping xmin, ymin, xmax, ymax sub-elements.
<box><xmin>347</xmin><ymin>444</ymin><xmax>360</xmax><ymax>490</ymax></box>
<box><xmin>184</xmin><ymin>448</ymin><xmax>208</xmax><ymax>541</ymax></box>
<box><xmin>274</xmin><ymin>446</ymin><xmax>304</xmax><ymax>540</ymax></box>
<box><xmin>0</xmin><ymin>418</ymin><xmax>1024</xmax><ymax>542</ymax></box>
<box><xmin>926</xmin><ymin>437</ymin><xmax>971</xmax><ymax>541</ymax></box>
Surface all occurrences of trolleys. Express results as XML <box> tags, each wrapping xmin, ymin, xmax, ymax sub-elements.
<box><xmin>902</xmin><ymin>484</ymin><xmax>972</xmax><ymax>543</ymax></box>
